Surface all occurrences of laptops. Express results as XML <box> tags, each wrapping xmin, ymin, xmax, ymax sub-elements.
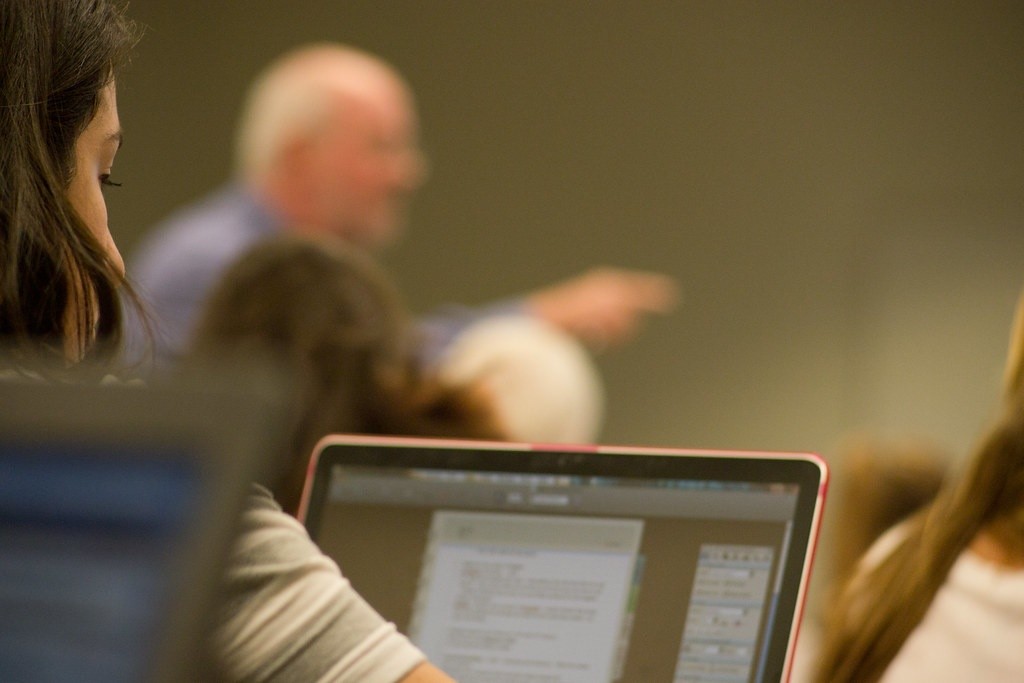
<box><xmin>0</xmin><ymin>361</ymin><xmax>286</xmax><ymax>683</ymax></box>
<box><xmin>297</xmin><ymin>434</ymin><xmax>831</xmax><ymax>683</ymax></box>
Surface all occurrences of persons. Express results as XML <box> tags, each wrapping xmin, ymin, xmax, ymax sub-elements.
<box><xmin>799</xmin><ymin>288</ymin><xmax>1024</xmax><ymax>683</ymax></box>
<box><xmin>123</xmin><ymin>44</ymin><xmax>674</xmax><ymax>391</ymax></box>
<box><xmin>0</xmin><ymin>0</ymin><xmax>460</xmax><ymax>683</ymax></box>
<box><xmin>185</xmin><ymin>233</ymin><xmax>504</xmax><ymax>523</ymax></box>
